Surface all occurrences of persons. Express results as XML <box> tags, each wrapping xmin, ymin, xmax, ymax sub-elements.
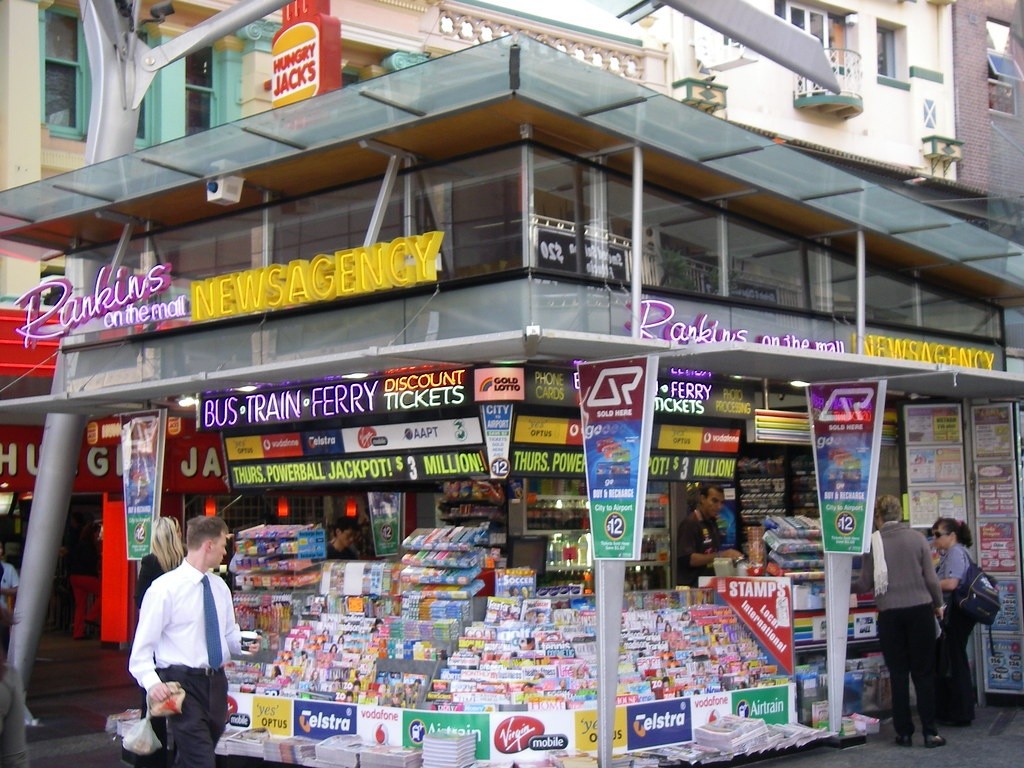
<box><xmin>931</xmin><ymin>517</ymin><xmax>980</xmax><ymax>726</ymax></box>
<box><xmin>128</xmin><ymin>513</ymin><xmax>263</xmax><ymax>768</ymax></box>
<box><xmin>324</xmin><ymin>516</ymin><xmax>359</xmax><ymax>559</ymax></box>
<box><xmin>51</xmin><ymin>512</ymin><xmax>103</xmax><ymax>641</ymax></box>
<box><xmin>859</xmin><ymin>496</ymin><xmax>946</xmax><ymax>748</ymax></box>
<box><xmin>0</xmin><ymin>539</ymin><xmax>37</xmax><ymax>768</ymax></box>
<box><xmin>675</xmin><ymin>483</ymin><xmax>745</xmax><ymax>588</ymax></box>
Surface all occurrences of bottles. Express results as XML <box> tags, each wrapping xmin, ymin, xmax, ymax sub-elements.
<box><xmin>550</xmin><ymin>569</ymin><xmax>594</xmax><ymax>596</ymax></box>
<box><xmin>527</xmin><ymin>500</ymin><xmax>590</xmax><ymax>529</ymax></box>
<box><xmin>548</xmin><ymin>534</ymin><xmax>588</xmax><ymax>566</ymax></box>
<box><xmin>643</xmin><ymin>499</ymin><xmax>667</xmax><ymax>529</ymax></box>
<box><xmin>625</xmin><ymin>566</ymin><xmax>666</xmax><ymax>592</ymax></box>
<box><xmin>641</xmin><ymin>534</ymin><xmax>669</xmax><ymax>562</ymax></box>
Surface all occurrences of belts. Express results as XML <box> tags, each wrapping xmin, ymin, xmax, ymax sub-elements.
<box><xmin>160</xmin><ymin>665</ymin><xmax>225</xmax><ymax>676</ymax></box>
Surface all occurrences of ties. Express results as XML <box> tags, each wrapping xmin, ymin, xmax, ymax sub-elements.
<box><xmin>201</xmin><ymin>575</ymin><xmax>222</xmax><ymax>672</ymax></box>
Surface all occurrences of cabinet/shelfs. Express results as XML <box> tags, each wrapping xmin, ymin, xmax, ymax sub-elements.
<box><xmin>225</xmin><ymin>451</ymin><xmax>919</xmax><ymax>729</ymax></box>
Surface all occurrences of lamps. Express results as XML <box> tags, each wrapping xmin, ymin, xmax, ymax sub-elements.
<box><xmin>346</xmin><ymin>496</ymin><xmax>357</xmax><ymax>517</ymax></box>
<box><xmin>277</xmin><ymin>496</ymin><xmax>288</xmax><ymax>516</ymax></box>
<box><xmin>205</xmin><ymin>494</ymin><xmax>216</xmax><ymax>516</ymax></box>
<box><xmin>139</xmin><ymin>0</ymin><xmax>176</xmax><ymax>27</ymax></box>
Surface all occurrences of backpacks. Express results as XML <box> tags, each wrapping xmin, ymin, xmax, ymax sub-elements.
<box><xmin>949</xmin><ymin>548</ymin><xmax>1001</xmax><ymax>626</ymax></box>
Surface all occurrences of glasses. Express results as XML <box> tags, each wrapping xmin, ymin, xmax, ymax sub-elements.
<box><xmin>931</xmin><ymin>531</ymin><xmax>949</xmax><ymax>538</ymax></box>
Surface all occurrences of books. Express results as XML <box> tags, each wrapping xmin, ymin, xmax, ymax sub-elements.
<box><xmin>215</xmin><ymin>565</ymin><xmax>890</xmax><ymax>767</ymax></box>
<box><xmin>105</xmin><ymin>708</ymin><xmax>141</xmax><ymax>738</ymax></box>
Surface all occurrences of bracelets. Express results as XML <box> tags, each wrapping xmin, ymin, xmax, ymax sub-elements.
<box><xmin>717</xmin><ymin>551</ymin><xmax>721</xmax><ymax>558</ymax></box>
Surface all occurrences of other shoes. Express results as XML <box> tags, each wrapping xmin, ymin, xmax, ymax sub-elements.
<box><xmin>896</xmin><ymin>735</ymin><xmax>912</xmax><ymax>747</ymax></box>
<box><xmin>924</xmin><ymin>735</ymin><xmax>946</xmax><ymax>748</ymax></box>
<box><xmin>939</xmin><ymin>718</ymin><xmax>971</xmax><ymax>727</ymax></box>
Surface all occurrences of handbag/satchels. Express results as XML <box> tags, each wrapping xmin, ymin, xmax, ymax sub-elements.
<box><xmin>123</xmin><ymin>697</ymin><xmax>161</xmax><ymax>755</ymax></box>
